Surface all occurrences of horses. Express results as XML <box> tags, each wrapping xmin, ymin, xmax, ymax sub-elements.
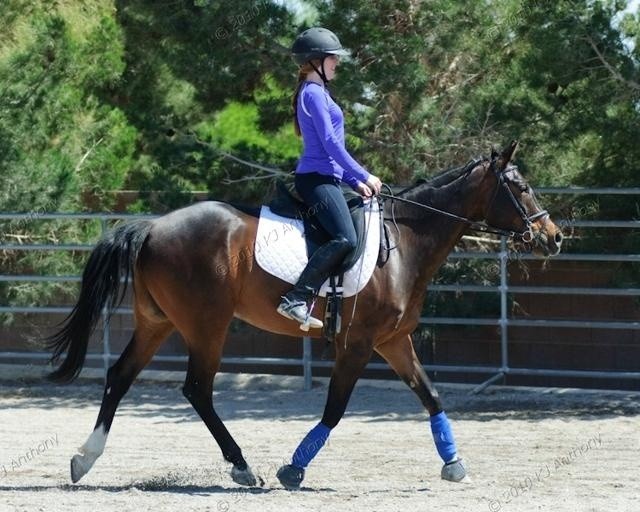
<box><xmin>42</xmin><ymin>141</ymin><xmax>566</xmax><ymax>488</ymax></box>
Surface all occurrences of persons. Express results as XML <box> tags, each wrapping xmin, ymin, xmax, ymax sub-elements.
<box><xmin>276</xmin><ymin>27</ymin><xmax>382</xmax><ymax>329</ymax></box>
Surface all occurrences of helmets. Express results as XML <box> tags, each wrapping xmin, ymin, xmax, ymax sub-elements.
<box><xmin>290</xmin><ymin>27</ymin><xmax>351</xmax><ymax>70</ymax></box>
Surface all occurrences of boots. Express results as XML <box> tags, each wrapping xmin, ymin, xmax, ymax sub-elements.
<box><xmin>276</xmin><ymin>236</ymin><xmax>356</xmax><ymax>329</ymax></box>
